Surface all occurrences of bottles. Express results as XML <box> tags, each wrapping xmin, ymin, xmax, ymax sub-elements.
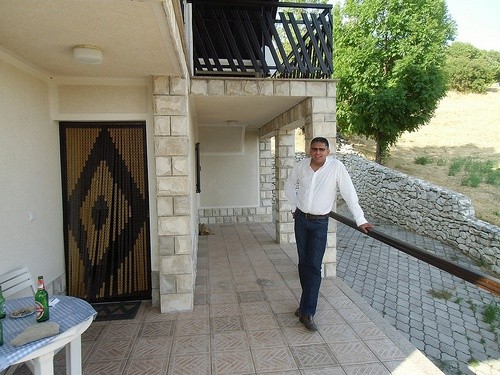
<box><xmin>0</xmin><ymin>285</ymin><xmax>6</xmax><ymax>319</ymax></box>
<box><xmin>0</xmin><ymin>317</ymin><xmax>4</xmax><ymax>346</ymax></box>
<box><xmin>35</xmin><ymin>276</ymin><xmax>49</xmax><ymax>322</ymax></box>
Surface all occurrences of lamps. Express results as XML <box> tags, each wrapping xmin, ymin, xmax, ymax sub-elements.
<box><xmin>73</xmin><ymin>44</ymin><xmax>103</xmax><ymax>64</ymax></box>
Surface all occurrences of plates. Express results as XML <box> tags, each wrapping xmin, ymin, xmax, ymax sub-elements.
<box><xmin>10</xmin><ymin>310</ymin><xmax>35</xmax><ymax>318</ymax></box>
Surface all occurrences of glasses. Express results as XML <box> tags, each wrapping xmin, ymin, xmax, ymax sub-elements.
<box><xmin>311</xmin><ymin>148</ymin><xmax>326</xmax><ymax>151</ymax></box>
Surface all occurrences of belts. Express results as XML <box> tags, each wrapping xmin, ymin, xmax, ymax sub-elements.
<box><xmin>297</xmin><ymin>208</ymin><xmax>328</xmax><ymax>220</ymax></box>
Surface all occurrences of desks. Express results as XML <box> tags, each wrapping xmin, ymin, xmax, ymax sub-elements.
<box><xmin>0</xmin><ymin>295</ymin><xmax>98</xmax><ymax>375</ymax></box>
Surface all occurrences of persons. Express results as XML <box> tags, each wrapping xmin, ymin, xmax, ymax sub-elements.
<box><xmin>284</xmin><ymin>137</ymin><xmax>371</xmax><ymax>330</ymax></box>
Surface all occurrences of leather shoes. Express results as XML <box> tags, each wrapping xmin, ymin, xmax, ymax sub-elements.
<box><xmin>295</xmin><ymin>308</ymin><xmax>301</xmax><ymax>315</ymax></box>
<box><xmin>299</xmin><ymin>316</ymin><xmax>317</xmax><ymax>330</ymax></box>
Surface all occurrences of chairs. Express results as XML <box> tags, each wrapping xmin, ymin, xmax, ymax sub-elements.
<box><xmin>0</xmin><ymin>267</ymin><xmax>37</xmax><ymax>300</ymax></box>
